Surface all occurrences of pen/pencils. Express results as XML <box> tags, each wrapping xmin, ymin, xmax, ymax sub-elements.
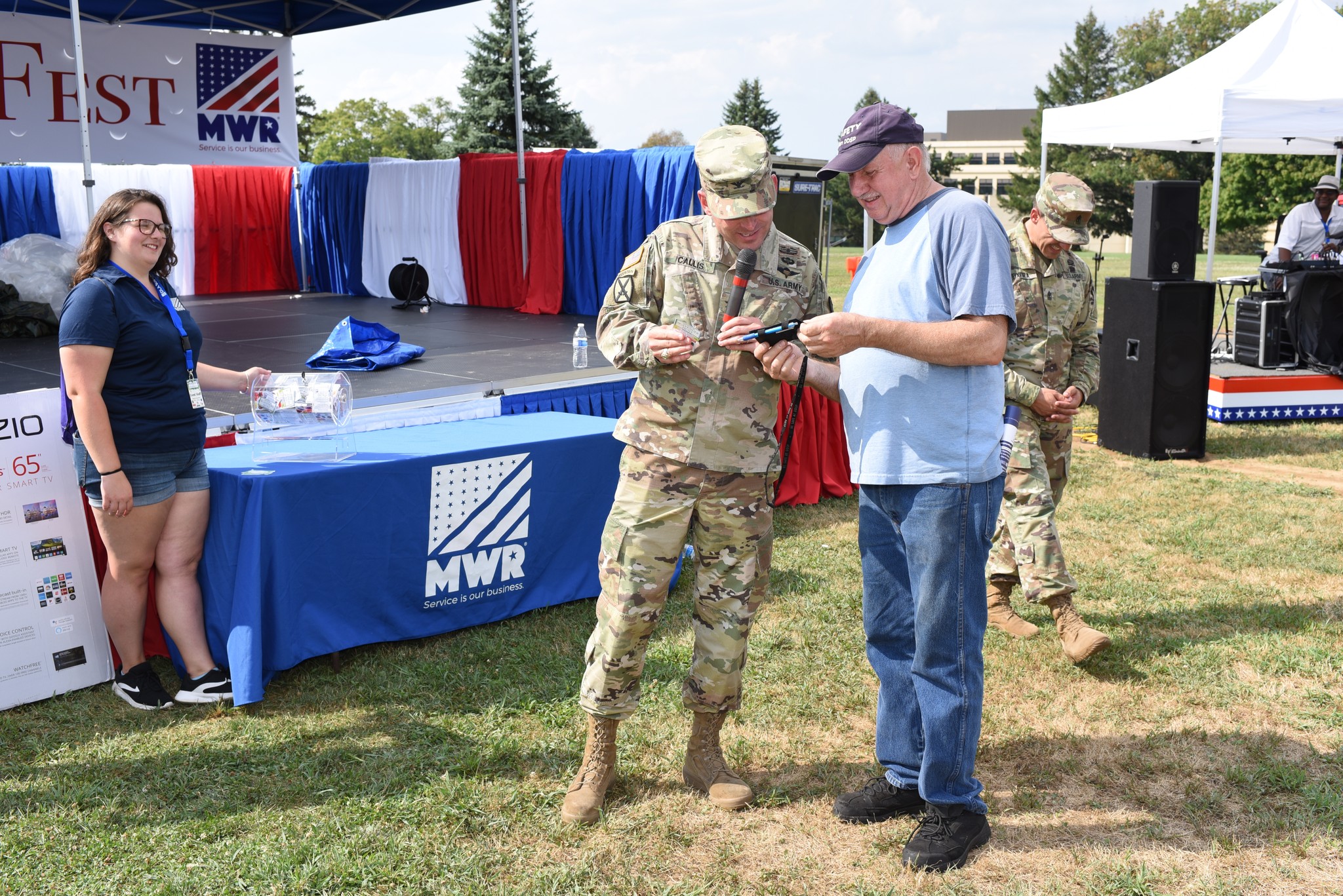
<box><xmin>737</xmin><ymin>322</ymin><xmax>798</xmax><ymax>341</ymax></box>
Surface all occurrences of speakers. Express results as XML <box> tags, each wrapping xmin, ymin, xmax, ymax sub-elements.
<box><xmin>1130</xmin><ymin>179</ymin><xmax>1203</xmax><ymax>281</ymax></box>
<box><xmin>1097</xmin><ymin>276</ymin><xmax>1217</xmax><ymax>461</ymax></box>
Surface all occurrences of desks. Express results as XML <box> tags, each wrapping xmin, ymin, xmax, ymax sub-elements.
<box><xmin>160</xmin><ymin>411</ymin><xmax>682</xmax><ymax>706</ymax></box>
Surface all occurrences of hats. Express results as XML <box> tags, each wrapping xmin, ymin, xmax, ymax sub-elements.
<box><xmin>816</xmin><ymin>101</ymin><xmax>925</xmax><ymax>180</ymax></box>
<box><xmin>1313</xmin><ymin>174</ymin><xmax>1339</xmax><ymax>189</ymax></box>
<box><xmin>1036</xmin><ymin>171</ymin><xmax>1095</xmax><ymax>245</ymax></box>
<box><xmin>694</xmin><ymin>125</ymin><xmax>777</xmax><ymax>219</ymax></box>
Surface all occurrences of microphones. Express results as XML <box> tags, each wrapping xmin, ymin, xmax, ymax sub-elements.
<box><xmin>719</xmin><ymin>248</ymin><xmax>757</xmax><ymax>342</ymax></box>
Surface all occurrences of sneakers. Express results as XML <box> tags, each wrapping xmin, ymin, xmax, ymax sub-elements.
<box><xmin>174</xmin><ymin>663</ymin><xmax>233</xmax><ymax>703</ymax></box>
<box><xmin>833</xmin><ymin>773</ymin><xmax>925</xmax><ymax>825</ymax></box>
<box><xmin>112</xmin><ymin>660</ymin><xmax>175</xmax><ymax>711</ymax></box>
<box><xmin>900</xmin><ymin>802</ymin><xmax>990</xmax><ymax>874</ymax></box>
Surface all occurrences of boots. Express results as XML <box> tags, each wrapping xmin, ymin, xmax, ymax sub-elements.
<box><xmin>560</xmin><ymin>713</ymin><xmax>618</xmax><ymax>828</ymax></box>
<box><xmin>986</xmin><ymin>581</ymin><xmax>1040</xmax><ymax>640</ymax></box>
<box><xmin>1044</xmin><ymin>593</ymin><xmax>1112</xmax><ymax>664</ymax></box>
<box><xmin>682</xmin><ymin>712</ymin><xmax>757</xmax><ymax>811</ymax></box>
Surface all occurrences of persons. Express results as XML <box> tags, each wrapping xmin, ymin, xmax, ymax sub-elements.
<box><xmin>1260</xmin><ymin>175</ymin><xmax>1342</xmax><ymax>293</ymax></box>
<box><xmin>984</xmin><ymin>174</ymin><xmax>1115</xmax><ymax>663</ymax></box>
<box><xmin>753</xmin><ymin>101</ymin><xmax>1016</xmax><ymax>870</ymax></box>
<box><xmin>562</xmin><ymin>122</ymin><xmax>838</xmax><ymax>823</ymax></box>
<box><xmin>98</xmin><ymin>468</ymin><xmax>123</xmax><ymax>476</ymax></box>
<box><xmin>56</xmin><ymin>186</ymin><xmax>269</xmax><ymax>709</ymax></box>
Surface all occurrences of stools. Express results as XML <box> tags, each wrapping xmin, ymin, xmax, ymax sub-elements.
<box><xmin>1211</xmin><ymin>279</ymin><xmax>1259</xmax><ymax>355</ymax></box>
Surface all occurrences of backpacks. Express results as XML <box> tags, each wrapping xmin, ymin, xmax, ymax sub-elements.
<box><xmin>60</xmin><ymin>275</ymin><xmax>116</xmax><ymax>446</ymax></box>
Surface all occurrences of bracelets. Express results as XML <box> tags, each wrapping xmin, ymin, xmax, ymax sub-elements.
<box><xmin>240</xmin><ymin>371</ymin><xmax>249</xmax><ymax>394</ymax></box>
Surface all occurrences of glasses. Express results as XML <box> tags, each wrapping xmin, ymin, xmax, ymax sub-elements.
<box><xmin>121</xmin><ymin>218</ymin><xmax>172</xmax><ymax>239</ymax></box>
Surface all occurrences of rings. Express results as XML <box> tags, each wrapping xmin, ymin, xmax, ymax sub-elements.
<box><xmin>661</xmin><ymin>348</ymin><xmax>671</xmax><ymax>360</ymax></box>
<box><xmin>747</xmin><ymin>340</ymin><xmax>749</xmax><ymax>343</ymax></box>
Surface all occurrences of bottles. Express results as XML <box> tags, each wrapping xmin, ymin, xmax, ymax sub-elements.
<box><xmin>682</xmin><ymin>543</ymin><xmax>695</xmax><ymax>560</ymax></box>
<box><xmin>573</xmin><ymin>323</ymin><xmax>587</xmax><ymax>368</ymax></box>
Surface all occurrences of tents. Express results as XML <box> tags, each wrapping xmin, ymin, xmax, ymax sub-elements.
<box><xmin>1</xmin><ymin>1</ymin><xmax>512</xmax><ymax>36</ymax></box>
<box><xmin>1044</xmin><ymin>0</ymin><xmax>1343</xmax><ymax>159</ymax></box>
